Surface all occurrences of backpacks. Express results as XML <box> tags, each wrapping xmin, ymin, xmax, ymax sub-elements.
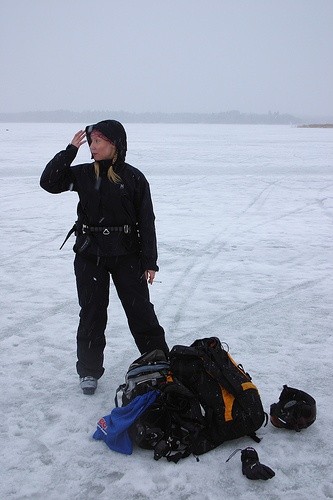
<box><xmin>114</xmin><ymin>336</ymin><xmax>269</xmax><ymax>464</ymax></box>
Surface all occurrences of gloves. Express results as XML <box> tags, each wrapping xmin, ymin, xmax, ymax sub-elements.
<box><xmin>225</xmin><ymin>447</ymin><xmax>276</xmax><ymax>480</ymax></box>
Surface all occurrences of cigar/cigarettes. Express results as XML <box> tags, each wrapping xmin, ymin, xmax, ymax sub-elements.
<box><xmin>148</xmin><ymin>280</ymin><xmax>162</xmax><ymax>284</ymax></box>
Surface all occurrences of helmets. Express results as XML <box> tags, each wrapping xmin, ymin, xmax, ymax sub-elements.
<box><xmin>269</xmin><ymin>384</ymin><xmax>317</xmax><ymax>432</ymax></box>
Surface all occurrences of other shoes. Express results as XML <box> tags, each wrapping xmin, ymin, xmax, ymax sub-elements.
<box><xmin>79</xmin><ymin>377</ymin><xmax>98</xmax><ymax>395</ymax></box>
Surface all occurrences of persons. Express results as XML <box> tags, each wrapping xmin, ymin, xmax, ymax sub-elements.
<box><xmin>39</xmin><ymin>119</ymin><xmax>170</xmax><ymax>395</ymax></box>
